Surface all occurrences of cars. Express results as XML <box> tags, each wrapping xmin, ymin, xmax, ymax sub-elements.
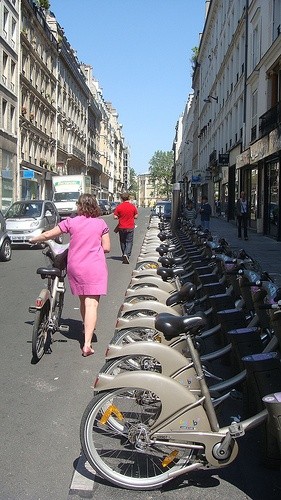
<box><xmin>96</xmin><ymin>199</ymin><xmax>121</xmax><ymax>215</ymax></box>
<box><xmin>4</xmin><ymin>199</ymin><xmax>50</xmax><ymax>251</ymax></box>
<box><xmin>0</xmin><ymin>209</ymin><xmax>13</xmax><ymax>262</ymax></box>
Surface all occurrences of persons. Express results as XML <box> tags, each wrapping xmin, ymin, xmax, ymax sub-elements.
<box><xmin>29</xmin><ymin>193</ymin><xmax>111</xmax><ymax>358</ymax></box>
<box><xmin>113</xmin><ymin>193</ymin><xmax>139</xmax><ymax>264</ymax></box>
<box><xmin>216</xmin><ymin>199</ymin><xmax>222</xmax><ymax>216</ymax></box>
<box><xmin>184</xmin><ymin>196</ymin><xmax>197</xmax><ymax>226</ymax></box>
<box><xmin>273</xmin><ymin>201</ymin><xmax>280</xmax><ymax>225</ymax></box>
<box><xmin>198</xmin><ymin>196</ymin><xmax>211</xmax><ymax>230</ymax></box>
<box><xmin>235</xmin><ymin>192</ymin><xmax>251</xmax><ymax>240</ymax></box>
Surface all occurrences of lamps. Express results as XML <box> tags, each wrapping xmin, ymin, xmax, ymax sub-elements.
<box><xmin>204</xmin><ymin>95</ymin><xmax>218</xmax><ymax>104</ymax></box>
<box><xmin>185</xmin><ymin>140</ymin><xmax>193</xmax><ymax>144</ymax></box>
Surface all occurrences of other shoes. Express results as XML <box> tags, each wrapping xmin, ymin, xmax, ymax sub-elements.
<box><xmin>82</xmin><ymin>346</ymin><xmax>93</xmax><ymax>357</ymax></box>
<box><xmin>238</xmin><ymin>237</ymin><xmax>242</xmax><ymax>240</ymax></box>
<box><xmin>122</xmin><ymin>253</ymin><xmax>130</xmax><ymax>264</ymax></box>
<box><xmin>244</xmin><ymin>236</ymin><xmax>249</xmax><ymax>240</ymax></box>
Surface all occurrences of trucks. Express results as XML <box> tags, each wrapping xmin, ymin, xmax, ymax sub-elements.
<box><xmin>51</xmin><ymin>174</ymin><xmax>92</xmax><ymax>221</ymax></box>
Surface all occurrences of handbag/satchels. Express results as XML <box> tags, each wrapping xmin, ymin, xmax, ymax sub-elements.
<box><xmin>242</xmin><ymin>213</ymin><xmax>249</xmax><ymax>221</ymax></box>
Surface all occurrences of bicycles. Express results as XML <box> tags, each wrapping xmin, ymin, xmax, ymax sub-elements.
<box><xmin>88</xmin><ymin>204</ymin><xmax>281</xmax><ymax>440</ymax></box>
<box><xmin>79</xmin><ymin>300</ymin><xmax>281</xmax><ymax>493</ymax></box>
<box><xmin>28</xmin><ymin>235</ymin><xmax>70</xmax><ymax>362</ymax></box>
